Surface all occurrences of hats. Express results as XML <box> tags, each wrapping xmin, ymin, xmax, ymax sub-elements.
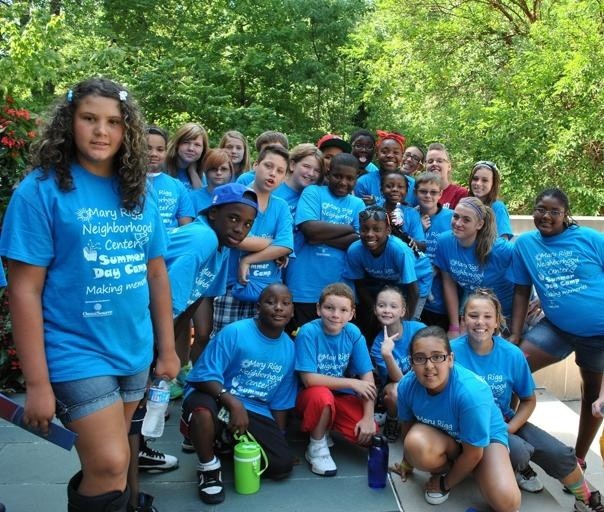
<box><xmin>316</xmin><ymin>135</ymin><xmax>353</xmax><ymax>154</ymax></box>
<box><xmin>196</xmin><ymin>183</ymin><xmax>259</xmax><ymax>216</ymax></box>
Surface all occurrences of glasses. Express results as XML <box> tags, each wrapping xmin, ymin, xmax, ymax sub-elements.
<box><xmin>409</xmin><ymin>353</ymin><xmax>449</xmax><ymax>365</ymax></box>
<box><xmin>532</xmin><ymin>205</ymin><xmax>562</xmax><ymax>220</ymax></box>
<box><xmin>415</xmin><ymin>189</ymin><xmax>441</xmax><ymax>197</ymax></box>
<box><xmin>403</xmin><ymin>151</ymin><xmax>422</xmax><ymax>164</ymax></box>
<box><xmin>358</xmin><ymin>209</ymin><xmax>387</xmax><ymax>222</ymax></box>
<box><xmin>351</xmin><ymin>143</ymin><xmax>376</xmax><ymax>151</ymax></box>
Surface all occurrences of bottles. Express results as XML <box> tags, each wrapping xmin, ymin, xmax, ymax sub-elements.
<box><xmin>141</xmin><ymin>371</ymin><xmax>170</xmax><ymax>439</ymax></box>
<box><xmin>366</xmin><ymin>433</ymin><xmax>390</xmax><ymax>490</ymax></box>
<box><xmin>235</xmin><ymin>433</ymin><xmax>263</xmax><ymax>493</ymax></box>
<box><xmin>390</xmin><ymin>201</ymin><xmax>405</xmax><ymax>226</ymax></box>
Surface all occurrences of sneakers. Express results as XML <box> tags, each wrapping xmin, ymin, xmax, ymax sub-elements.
<box><xmin>571</xmin><ymin>488</ymin><xmax>603</xmax><ymax>512</ymax></box>
<box><xmin>561</xmin><ymin>456</ymin><xmax>587</xmax><ymax>493</ymax></box>
<box><xmin>383</xmin><ymin>414</ymin><xmax>401</xmax><ymax>443</ymax></box>
<box><xmin>512</xmin><ymin>464</ymin><xmax>544</xmax><ymax>493</ymax></box>
<box><xmin>148</xmin><ymin>368</ymin><xmax>183</xmax><ymax>400</ymax></box>
<box><xmin>303</xmin><ymin>426</ymin><xmax>337</xmax><ymax>476</ymax></box>
<box><xmin>137</xmin><ymin>435</ymin><xmax>178</xmax><ymax>474</ymax></box>
<box><xmin>195</xmin><ymin>456</ymin><xmax>225</xmax><ymax>504</ymax></box>
<box><xmin>181</xmin><ymin>437</ymin><xmax>196</xmax><ymax>452</ymax></box>
<box><xmin>373</xmin><ymin>410</ymin><xmax>387</xmax><ymax>426</ymax></box>
<box><xmin>424</xmin><ymin>470</ymin><xmax>452</xmax><ymax>504</ymax></box>
<box><xmin>131</xmin><ymin>405</ymin><xmax>169</xmax><ymax>434</ymax></box>
<box><xmin>172</xmin><ymin>360</ymin><xmax>192</xmax><ymax>386</ymax></box>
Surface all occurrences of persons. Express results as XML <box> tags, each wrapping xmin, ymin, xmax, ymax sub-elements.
<box><xmin>388</xmin><ymin>320</ymin><xmax>526</xmax><ymax>511</ymax></box>
<box><xmin>124</xmin><ymin>124</ymin><xmax>526</xmax><ymax>502</ymax></box>
<box><xmin>508</xmin><ymin>186</ymin><xmax>603</xmax><ymax>472</ymax></box>
<box><xmin>442</xmin><ymin>289</ymin><xmax>603</xmax><ymax>510</ymax></box>
<box><xmin>0</xmin><ymin>71</ymin><xmax>179</xmax><ymax>511</ymax></box>
